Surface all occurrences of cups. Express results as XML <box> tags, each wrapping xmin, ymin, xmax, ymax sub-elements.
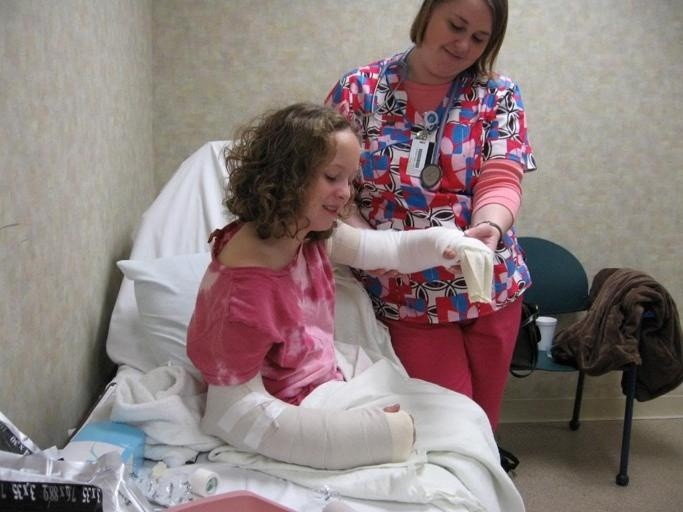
<box><xmin>536</xmin><ymin>315</ymin><xmax>558</xmax><ymax>351</ymax></box>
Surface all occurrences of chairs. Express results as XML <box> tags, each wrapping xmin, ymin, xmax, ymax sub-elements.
<box><xmin>499</xmin><ymin>235</ymin><xmax>644</xmax><ymax>487</ymax></box>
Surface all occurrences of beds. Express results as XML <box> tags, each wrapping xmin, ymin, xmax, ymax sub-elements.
<box><xmin>52</xmin><ymin>137</ymin><xmax>483</xmax><ymax>512</ymax></box>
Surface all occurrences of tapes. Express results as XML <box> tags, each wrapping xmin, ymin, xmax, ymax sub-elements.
<box><xmin>190</xmin><ymin>468</ymin><xmax>220</xmax><ymax>497</ymax></box>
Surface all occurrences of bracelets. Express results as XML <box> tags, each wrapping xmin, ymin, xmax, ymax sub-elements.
<box><xmin>474</xmin><ymin>220</ymin><xmax>503</xmax><ymax>242</ymax></box>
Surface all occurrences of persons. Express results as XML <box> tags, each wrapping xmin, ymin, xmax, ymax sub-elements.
<box><xmin>186</xmin><ymin>98</ymin><xmax>492</xmax><ymax>471</ymax></box>
<box><xmin>319</xmin><ymin>0</ymin><xmax>536</xmax><ymax>430</ymax></box>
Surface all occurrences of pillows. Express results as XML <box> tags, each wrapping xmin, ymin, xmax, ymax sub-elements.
<box><xmin>119</xmin><ymin>248</ymin><xmax>390</xmax><ymax>372</ymax></box>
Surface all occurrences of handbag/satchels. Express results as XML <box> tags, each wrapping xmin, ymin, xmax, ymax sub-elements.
<box><xmin>512</xmin><ymin>302</ymin><xmax>540</xmax><ymax>368</ymax></box>
